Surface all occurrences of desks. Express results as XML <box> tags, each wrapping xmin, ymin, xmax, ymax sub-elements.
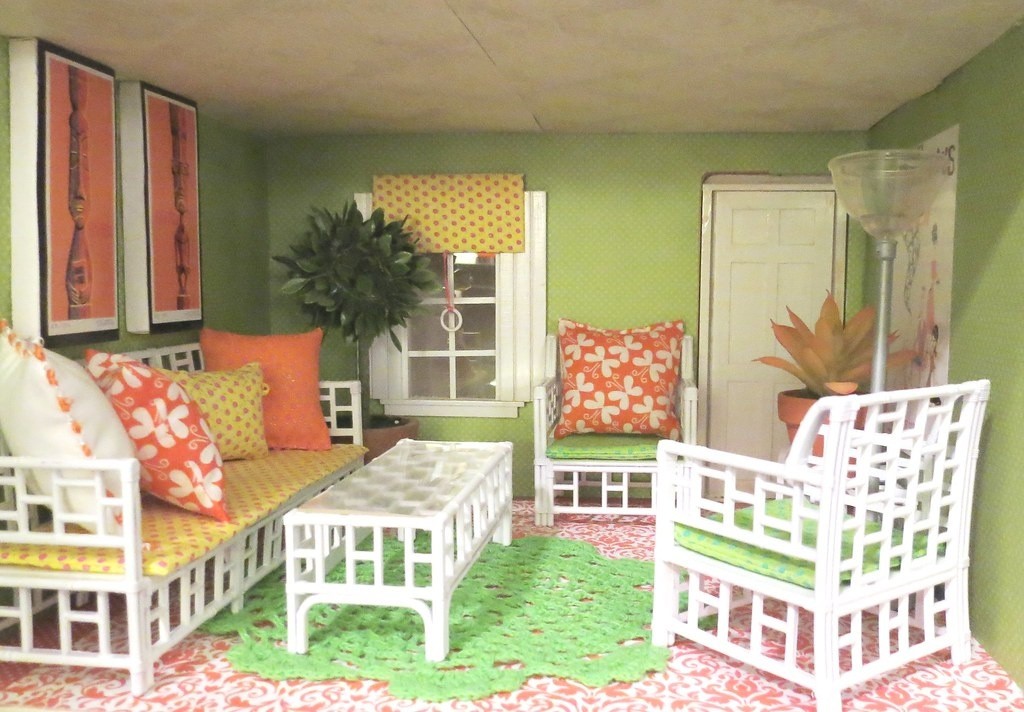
<box><xmin>280</xmin><ymin>435</ymin><xmax>514</xmax><ymax>667</ymax></box>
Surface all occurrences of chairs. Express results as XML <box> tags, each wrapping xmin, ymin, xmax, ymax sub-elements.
<box><xmin>533</xmin><ymin>332</ymin><xmax>699</xmax><ymax>528</ymax></box>
<box><xmin>649</xmin><ymin>379</ymin><xmax>991</xmax><ymax>712</ymax></box>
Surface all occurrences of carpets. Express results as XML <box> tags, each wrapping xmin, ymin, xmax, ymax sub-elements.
<box><xmin>191</xmin><ymin>528</ymin><xmax>720</xmax><ymax>704</ymax></box>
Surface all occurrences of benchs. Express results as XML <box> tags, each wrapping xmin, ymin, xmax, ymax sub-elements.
<box><xmin>0</xmin><ymin>341</ymin><xmax>365</xmax><ymax>699</ymax></box>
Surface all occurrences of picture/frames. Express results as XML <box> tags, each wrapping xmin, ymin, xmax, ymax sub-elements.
<box><xmin>119</xmin><ymin>77</ymin><xmax>205</xmax><ymax>336</ymax></box>
<box><xmin>8</xmin><ymin>37</ymin><xmax>121</xmax><ymax>349</ymax></box>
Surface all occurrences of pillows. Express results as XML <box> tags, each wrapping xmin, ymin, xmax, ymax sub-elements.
<box><xmin>85</xmin><ymin>348</ymin><xmax>232</xmax><ymax>524</ymax></box>
<box><xmin>0</xmin><ymin>317</ymin><xmax>142</xmax><ymax>538</ymax></box>
<box><xmin>198</xmin><ymin>326</ymin><xmax>335</xmax><ymax>452</ymax></box>
<box><xmin>554</xmin><ymin>317</ymin><xmax>686</xmax><ymax>444</ymax></box>
<box><xmin>152</xmin><ymin>360</ymin><xmax>272</xmax><ymax>463</ymax></box>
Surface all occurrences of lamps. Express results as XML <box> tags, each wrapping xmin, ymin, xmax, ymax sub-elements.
<box><xmin>827</xmin><ymin>148</ymin><xmax>950</xmax><ymax>526</ymax></box>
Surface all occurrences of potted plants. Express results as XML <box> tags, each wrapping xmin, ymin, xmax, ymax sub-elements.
<box><xmin>753</xmin><ymin>296</ymin><xmax>916</xmax><ymax>480</ymax></box>
<box><xmin>268</xmin><ymin>198</ymin><xmax>441</xmax><ymax>464</ymax></box>
